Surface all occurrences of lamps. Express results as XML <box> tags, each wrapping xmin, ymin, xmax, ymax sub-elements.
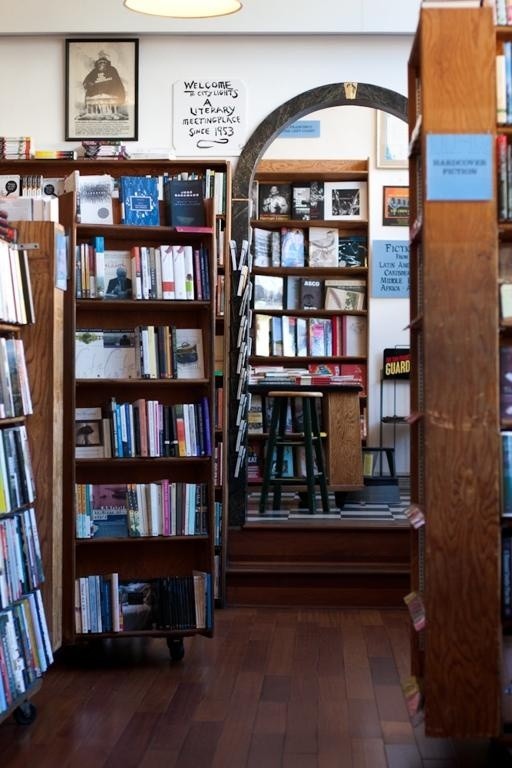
<box><xmin>121</xmin><ymin>0</ymin><xmax>243</xmax><ymax>19</ymax></box>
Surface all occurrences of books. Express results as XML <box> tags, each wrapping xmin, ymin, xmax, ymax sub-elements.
<box><xmin>490</xmin><ymin>2</ymin><xmax>512</xmax><ymax>626</ymax></box>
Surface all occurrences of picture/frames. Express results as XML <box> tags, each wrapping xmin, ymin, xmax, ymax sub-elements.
<box><xmin>63</xmin><ymin>37</ymin><xmax>139</xmax><ymax>143</ymax></box>
<box><xmin>382</xmin><ymin>185</ymin><xmax>410</xmax><ymax>226</ymax></box>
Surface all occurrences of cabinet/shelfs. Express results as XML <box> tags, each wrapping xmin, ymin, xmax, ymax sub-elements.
<box><xmin>0</xmin><ymin>220</ymin><xmax>62</xmax><ymax>726</ymax></box>
<box><xmin>242</xmin><ymin>158</ymin><xmax>370</xmax><ymax>487</ymax></box>
<box><xmin>403</xmin><ymin>3</ymin><xmax>512</xmax><ymax>763</ymax></box>
<box><xmin>4</xmin><ymin>159</ymin><xmax>228</xmax><ymax>661</ymax></box>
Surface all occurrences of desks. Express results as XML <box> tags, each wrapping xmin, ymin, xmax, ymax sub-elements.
<box><xmin>247</xmin><ymin>377</ymin><xmax>366</xmax><ymax>509</ymax></box>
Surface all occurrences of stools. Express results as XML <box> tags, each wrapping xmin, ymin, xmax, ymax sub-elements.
<box><xmin>258</xmin><ymin>389</ymin><xmax>331</xmax><ymax>514</ymax></box>
